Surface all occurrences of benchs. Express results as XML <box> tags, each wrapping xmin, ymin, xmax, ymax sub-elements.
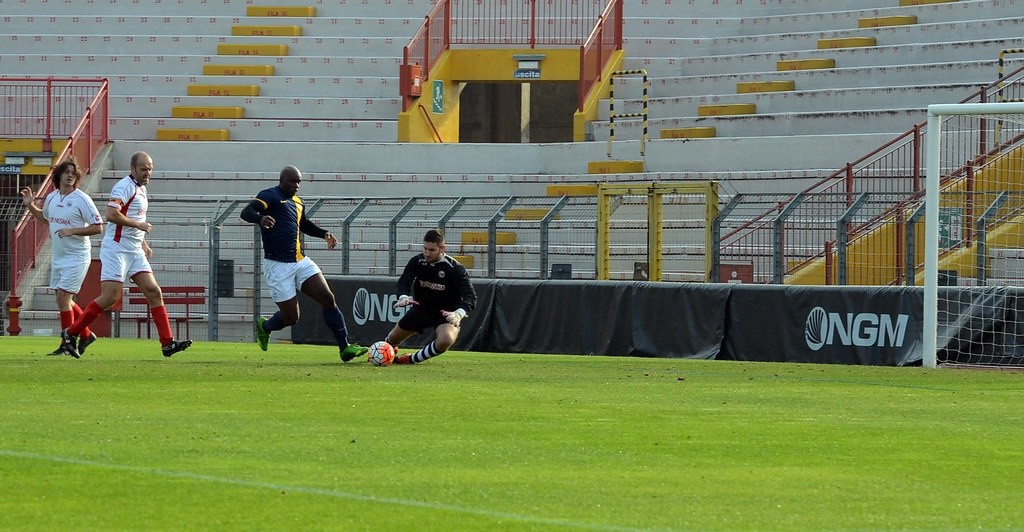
<box><xmin>129</xmin><ymin>286</ymin><xmax>205</xmax><ymax>340</ymax></box>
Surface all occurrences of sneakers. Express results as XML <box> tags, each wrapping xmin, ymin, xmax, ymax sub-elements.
<box><xmin>256</xmin><ymin>317</ymin><xmax>271</xmax><ymax>351</ymax></box>
<box><xmin>392</xmin><ymin>353</ymin><xmax>415</xmax><ymax>364</ymax></box>
<box><xmin>367</xmin><ymin>347</ymin><xmax>399</xmax><ymax>363</ymax></box>
<box><xmin>162</xmin><ymin>339</ymin><xmax>192</xmax><ymax>357</ymax></box>
<box><xmin>47</xmin><ymin>344</ymin><xmax>70</xmax><ymax>355</ymax></box>
<box><xmin>339</xmin><ymin>343</ymin><xmax>369</xmax><ymax>363</ymax></box>
<box><xmin>61</xmin><ymin>328</ymin><xmax>81</xmax><ymax>359</ymax></box>
<box><xmin>78</xmin><ymin>332</ymin><xmax>96</xmax><ymax>355</ymax></box>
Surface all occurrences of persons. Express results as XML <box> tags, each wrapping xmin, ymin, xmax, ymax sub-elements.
<box><xmin>239</xmin><ymin>165</ymin><xmax>368</xmax><ymax>363</ymax></box>
<box><xmin>20</xmin><ymin>155</ymin><xmax>103</xmax><ymax>356</ymax></box>
<box><xmin>366</xmin><ymin>229</ymin><xmax>477</xmax><ymax>364</ymax></box>
<box><xmin>61</xmin><ymin>151</ymin><xmax>193</xmax><ymax>358</ymax></box>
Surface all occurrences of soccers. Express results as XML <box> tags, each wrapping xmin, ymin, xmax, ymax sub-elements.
<box><xmin>367</xmin><ymin>341</ymin><xmax>396</xmax><ymax>367</ymax></box>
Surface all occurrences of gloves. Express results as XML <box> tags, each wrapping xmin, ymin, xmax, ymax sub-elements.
<box><xmin>393</xmin><ymin>295</ymin><xmax>419</xmax><ymax>308</ymax></box>
<box><xmin>440</xmin><ymin>308</ymin><xmax>469</xmax><ymax>327</ymax></box>
